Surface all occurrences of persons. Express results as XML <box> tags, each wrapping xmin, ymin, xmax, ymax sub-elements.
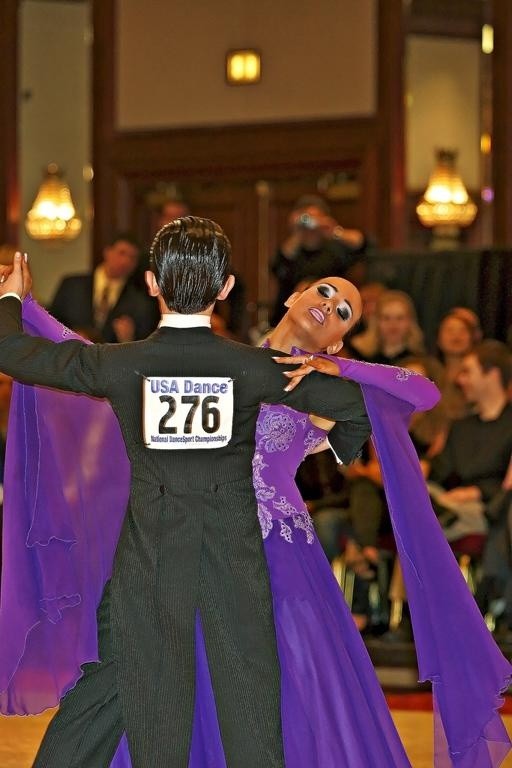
<box><xmin>0</xmin><ymin>249</ymin><xmax>443</xmax><ymax>767</ymax></box>
<box><xmin>2</xmin><ymin>215</ymin><xmax>375</xmax><ymax>767</ymax></box>
<box><xmin>282</xmin><ymin>255</ymin><xmax>512</xmax><ymax>640</ymax></box>
<box><xmin>46</xmin><ymin>199</ymin><xmax>246</xmax><ymax>343</ymax></box>
<box><xmin>270</xmin><ymin>198</ymin><xmax>367</xmax><ymax>327</ymax></box>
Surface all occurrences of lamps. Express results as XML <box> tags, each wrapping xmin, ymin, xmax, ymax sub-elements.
<box><xmin>415</xmin><ymin>144</ymin><xmax>478</xmax><ymax>243</ymax></box>
<box><xmin>25</xmin><ymin>161</ymin><xmax>81</xmax><ymax>241</ymax></box>
<box><xmin>225</xmin><ymin>42</ymin><xmax>262</xmax><ymax>87</ymax></box>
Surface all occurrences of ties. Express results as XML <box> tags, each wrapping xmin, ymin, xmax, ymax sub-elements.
<box><xmin>94</xmin><ymin>283</ymin><xmax>111</xmax><ymax>326</ymax></box>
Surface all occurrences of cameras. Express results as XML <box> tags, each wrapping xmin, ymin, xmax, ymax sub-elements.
<box><xmin>297</xmin><ymin>212</ymin><xmax>318</xmax><ymax>230</ymax></box>
